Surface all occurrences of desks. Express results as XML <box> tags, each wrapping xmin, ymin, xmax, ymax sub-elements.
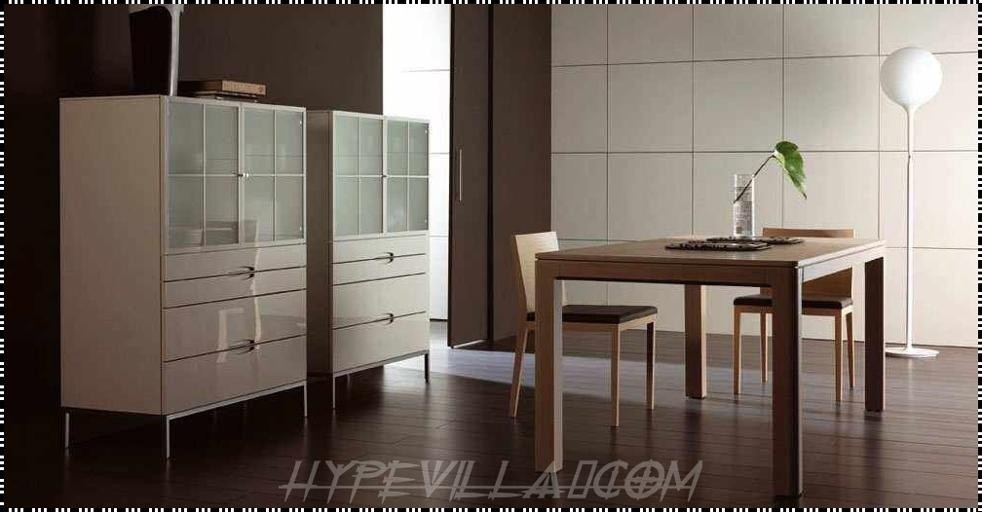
<box><xmin>531</xmin><ymin>236</ymin><xmax>888</xmax><ymax>494</ymax></box>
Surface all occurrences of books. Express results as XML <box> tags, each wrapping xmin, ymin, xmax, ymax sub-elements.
<box><xmin>178</xmin><ymin>80</ymin><xmax>267</xmax><ymax>102</ymax></box>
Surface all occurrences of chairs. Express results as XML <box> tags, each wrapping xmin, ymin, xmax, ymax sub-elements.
<box><xmin>730</xmin><ymin>226</ymin><xmax>857</xmax><ymax>401</ymax></box>
<box><xmin>507</xmin><ymin>230</ymin><xmax>659</xmax><ymax>428</ymax></box>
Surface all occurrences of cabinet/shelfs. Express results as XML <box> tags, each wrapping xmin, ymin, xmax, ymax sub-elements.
<box><xmin>58</xmin><ymin>93</ymin><xmax>432</xmax><ymax>460</ymax></box>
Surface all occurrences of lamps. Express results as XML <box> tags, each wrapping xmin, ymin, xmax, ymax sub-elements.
<box><xmin>880</xmin><ymin>44</ymin><xmax>944</xmax><ymax>360</ymax></box>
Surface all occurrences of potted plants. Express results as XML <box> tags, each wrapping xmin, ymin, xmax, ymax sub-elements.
<box><xmin>732</xmin><ymin>140</ymin><xmax>808</xmax><ymax>238</ymax></box>
